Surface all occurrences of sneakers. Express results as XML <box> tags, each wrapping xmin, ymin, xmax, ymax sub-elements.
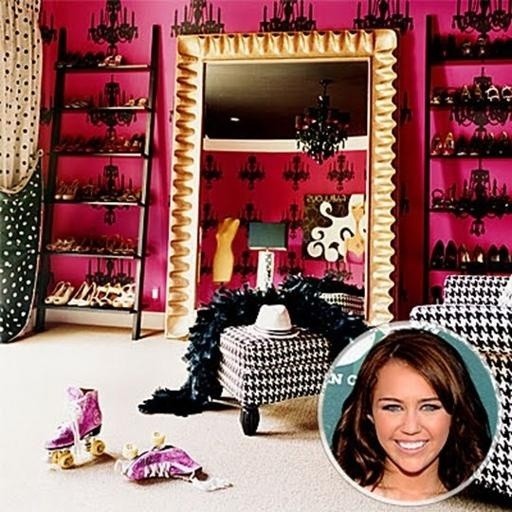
<box><xmin>430</xmin><ymin>82</ymin><xmax>512</xmax><ymax>105</ymax></box>
<box><xmin>432</xmin><ymin>34</ymin><xmax>512</xmax><ymax>59</ymax></box>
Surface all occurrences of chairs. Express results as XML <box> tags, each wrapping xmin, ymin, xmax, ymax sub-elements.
<box><xmin>416</xmin><ymin>276</ymin><xmax>511</xmax><ymax>498</ymax></box>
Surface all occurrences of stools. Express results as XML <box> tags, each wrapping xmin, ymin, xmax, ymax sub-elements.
<box><xmin>217</xmin><ymin>321</ymin><xmax>342</xmax><ymax>435</ymax></box>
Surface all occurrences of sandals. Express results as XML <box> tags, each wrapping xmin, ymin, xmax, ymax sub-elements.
<box><xmin>44</xmin><ymin>280</ymin><xmax>136</xmax><ymax>309</ymax></box>
<box><xmin>55</xmin><ymin>179</ymin><xmax>141</xmax><ymax>202</ymax></box>
<box><xmin>65</xmin><ymin>95</ymin><xmax>92</xmax><ymax>109</ymax></box>
<box><xmin>46</xmin><ymin>234</ymin><xmax>135</xmax><ymax>256</ymax></box>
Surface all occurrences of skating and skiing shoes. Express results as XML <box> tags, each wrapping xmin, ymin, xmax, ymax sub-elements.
<box><xmin>115</xmin><ymin>432</ymin><xmax>202</xmax><ymax>482</ymax></box>
<box><xmin>45</xmin><ymin>387</ymin><xmax>105</xmax><ymax>468</ymax></box>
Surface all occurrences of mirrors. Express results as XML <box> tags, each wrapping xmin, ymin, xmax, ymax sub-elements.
<box><xmin>164</xmin><ymin>29</ymin><xmax>399</xmax><ymax>328</ymax></box>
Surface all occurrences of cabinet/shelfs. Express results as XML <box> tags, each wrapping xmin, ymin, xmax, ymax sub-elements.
<box><xmin>421</xmin><ymin>13</ymin><xmax>512</xmax><ymax>303</ymax></box>
<box><xmin>34</xmin><ymin>20</ymin><xmax>161</xmax><ymax>343</ymax></box>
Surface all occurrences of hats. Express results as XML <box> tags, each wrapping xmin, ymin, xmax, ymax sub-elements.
<box><xmin>248</xmin><ymin>304</ymin><xmax>301</xmax><ymax>339</ymax></box>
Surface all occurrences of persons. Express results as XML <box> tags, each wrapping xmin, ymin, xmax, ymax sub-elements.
<box><xmin>327</xmin><ymin>329</ymin><xmax>492</xmax><ymax>505</ymax></box>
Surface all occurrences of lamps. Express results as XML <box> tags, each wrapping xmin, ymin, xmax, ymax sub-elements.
<box><xmin>294</xmin><ymin>78</ymin><xmax>352</xmax><ymax>167</ymax></box>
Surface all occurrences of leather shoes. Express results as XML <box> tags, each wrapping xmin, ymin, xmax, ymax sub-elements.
<box><xmin>54</xmin><ymin>134</ymin><xmax>145</xmax><ymax>153</ymax></box>
<box><xmin>430</xmin><ymin>132</ymin><xmax>512</xmax><ymax>156</ymax></box>
<box><xmin>57</xmin><ymin>52</ymin><xmax>122</xmax><ymax>68</ymax></box>
<box><xmin>431</xmin><ymin>240</ymin><xmax>510</xmax><ymax>274</ymax></box>
<box><xmin>431</xmin><ymin>287</ymin><xmax>443</xmax><ymax>304</ymax></box>
<box><xmin>432</xmin><ymin>188</ymin><xmax>511</xmax><ymax>211</ymax></box>
